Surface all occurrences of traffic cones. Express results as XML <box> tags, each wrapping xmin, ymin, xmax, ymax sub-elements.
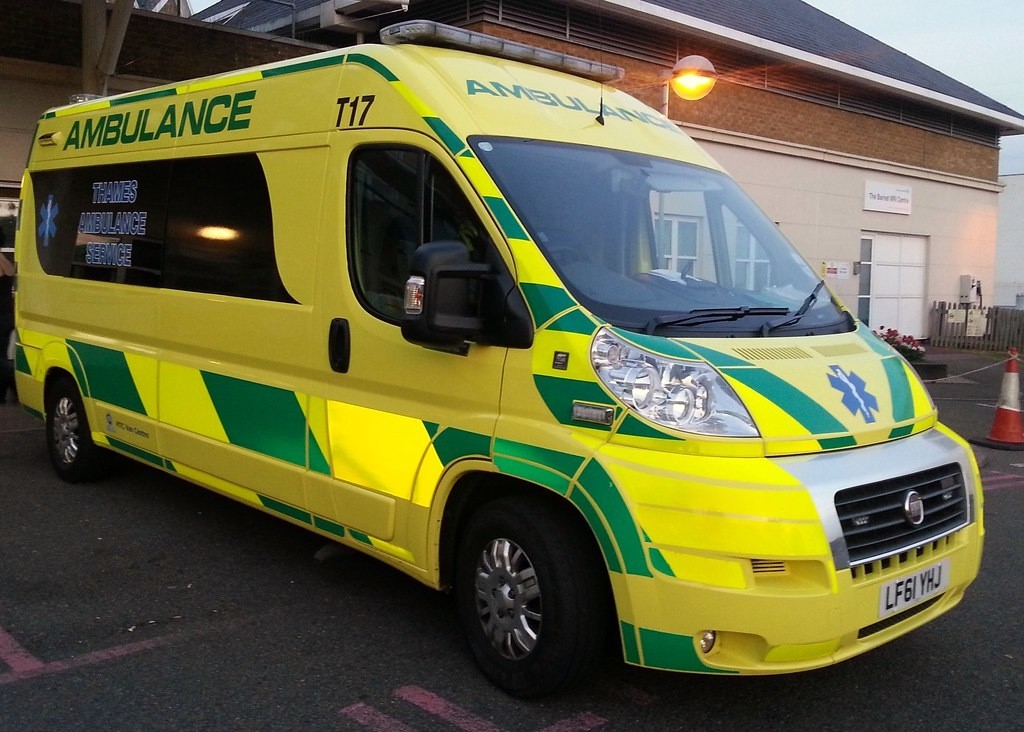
<box><xmin>968</xmin><ymin>347</ymin><xmax>1024</xmax><ymax>450</ymax></box>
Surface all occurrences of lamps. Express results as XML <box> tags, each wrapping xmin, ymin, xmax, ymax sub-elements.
<box><xmin>670</xmin><ymin>54</ymin><xmax>718</xmax><ymax>101</ymax></box>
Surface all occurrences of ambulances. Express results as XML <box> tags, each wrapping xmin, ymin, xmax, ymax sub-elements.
<box><xmin>12</xmin><ymin>19</ymin><xmax>988</xmax><ymax>708</ymax></box>
